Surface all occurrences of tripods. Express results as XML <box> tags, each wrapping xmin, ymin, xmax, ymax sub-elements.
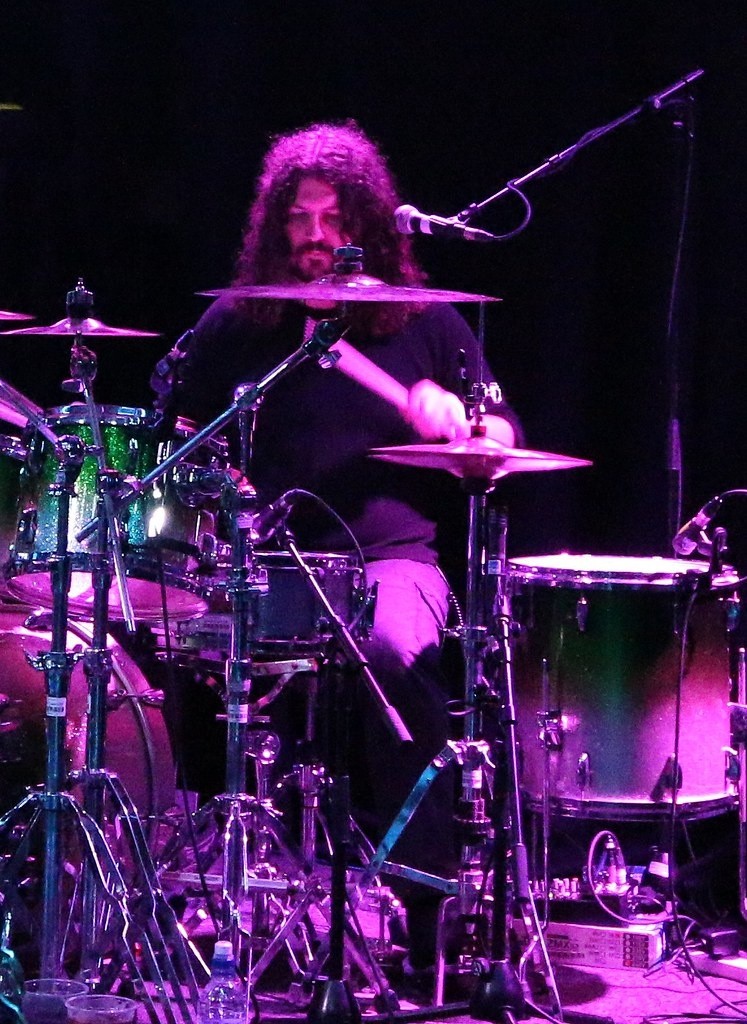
<box><xmin>0</xmin><ymin>303</ymin><xmax>612</xmax><ymax>1024</ymax></box>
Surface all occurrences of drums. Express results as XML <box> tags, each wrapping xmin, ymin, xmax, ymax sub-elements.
<box><xmin>7</xmin><ymin>401</ymin><xmax>233</xmax><ymax>622</ymax></box>
<box><xmin>0</xmin><ymin>614</ymin><xmax>179</xmax><ymax>967</ymax></box>
<box><xmin>196</xmin><ymin>544</ymin><xmax>363</xmax><ymax>654</ymax></box>
<box><xmin>498</xmin><ymin>548</ymin><xmax>744</xmax><ymax>823</ymax></box>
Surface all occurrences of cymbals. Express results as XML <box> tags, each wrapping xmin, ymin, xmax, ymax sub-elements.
<box><xmin>372</xmin><ymin>436</ymin><xmax>592</xmax><ymax>480</ymax></box>
<box><xmin>2</xmin><ymin>316</ymin><xmax>160</xmax><ymax>340</ymax></box>
<box><xmin>195</xmin><ymin>271</ymin><xmax>502</xmax><ymax>305</ymax></box>
<box><xmin>0</xmin><ymin>309</ymin><xmax>32</xmax><ymax>322</ymax></box>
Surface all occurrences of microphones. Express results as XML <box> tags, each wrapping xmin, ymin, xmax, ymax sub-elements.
<box><xmin>390</xmin><ymin>203</ymin><xmax>494</xmax><ymax>245</ymax></box>
<box><xmin>671</xmin><ymin>495</ymin><xmax>723</xmax><ymax>556</ymax></box>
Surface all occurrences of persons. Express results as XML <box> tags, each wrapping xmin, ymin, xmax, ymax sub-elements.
<box><xmin>132</xmin><ymin>125</ymin><xmax>526</xmax><ymax>998</ymax></box>
